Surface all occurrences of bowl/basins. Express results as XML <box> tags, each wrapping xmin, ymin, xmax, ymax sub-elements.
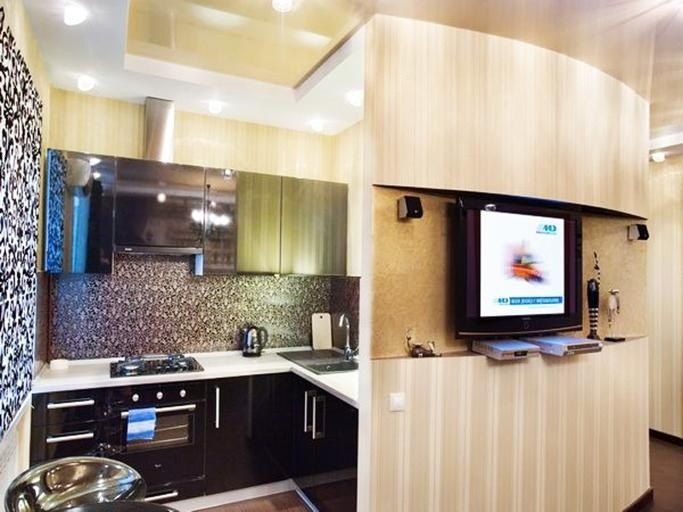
<box><xmin>5</xmin><ymin>453</ymin><xmax>179</xmax><ymax>511</ymax></box>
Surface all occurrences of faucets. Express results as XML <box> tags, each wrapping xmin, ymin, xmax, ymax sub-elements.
<box><xmin>338</xmin><ymin>312</ymin><xmax>352</xmax><ymax>353</ymax></box>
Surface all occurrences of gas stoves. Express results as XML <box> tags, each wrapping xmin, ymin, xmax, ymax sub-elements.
<box><xmin>105</xmin><ymin>352</ymin><xmax>204</xmax><ymax>407</ymax></box>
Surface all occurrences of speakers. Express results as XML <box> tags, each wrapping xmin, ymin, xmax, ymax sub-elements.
<box><xmin>628</xmin><ymin>224</ymin><xmax>649</xmax><ymax>240</ymax></box>
<box><xmin>398</xmin><ymin>196</ymin><xmax>423</xmax><ymax>219</ymax></box>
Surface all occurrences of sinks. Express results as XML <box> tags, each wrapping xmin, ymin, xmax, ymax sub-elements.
<box><xmin>278</xmin><ymin>349</ymin><xmax>344</xmax><ymax>362</ymax></box>
<box><xmin>306</xmin><ymin>360</ymin><xmax>359</xmax><ymax>374</ymax></box>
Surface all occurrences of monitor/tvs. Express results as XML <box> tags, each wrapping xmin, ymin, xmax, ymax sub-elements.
<box><xmin>456</xmin><ymin>195</ymin><xmax>582</xmax><ymax>336</ymax></box>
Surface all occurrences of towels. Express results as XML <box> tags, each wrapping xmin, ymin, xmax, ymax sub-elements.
<box><xmin>127</xmin><ymin>402</ymin><xmax>158</xmax><ymax>442</ymax></box>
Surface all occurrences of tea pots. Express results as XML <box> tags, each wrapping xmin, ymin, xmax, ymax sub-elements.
<box><xmin>310</xmin><ymin>312</ymin><xmax>332</xmax><ymax>350</ymax></box>
<box><xmin>237</xmin><ymin>324</ymin><xmax>269</xmax><ymax>357</ymax></box>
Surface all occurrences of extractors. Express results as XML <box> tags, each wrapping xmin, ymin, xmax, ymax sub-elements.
<box><xmin>114</xmin><ymin>243</ymin><xmax>203</xmax><ymax>258</ymax></box>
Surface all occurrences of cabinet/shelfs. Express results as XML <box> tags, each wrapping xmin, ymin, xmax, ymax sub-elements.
<box><xmin>206</xmin><ymin>374</ymin><xmax>252</xmax><ymax>494</ymax></box>
<box><xmin>253</xmin><ymin>368</ymin><xmax>294</xmax><ymax>486</ymax></box>
<box><xmin>195</xmin><ymin>166</ymin><xmax>237</xmax><ymax>276</ymax></box>
<box><xmin>30</xmin><ymin>389</ymin><xmax>107</xmax><ymax>466</ymax></box>
<box><xmin>237</xmin><ymin>169</ymin><xmax>282</xmax><ymax>274</ymax></box>
<box><xmin>281</xmin><ymin>175</ymin><xmax>348</xmax><ymax>279</ymax></box>
<box><xmin>44</xmin><ymin>148</ymin><xmax>115</xmax><ymax>275</ymax></box>
<box><xmin>295</xmin><ymin>374</ymin><xmax>358</xmax><ymax>512</ymax></box>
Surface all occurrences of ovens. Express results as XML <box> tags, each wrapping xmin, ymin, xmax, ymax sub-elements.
<box><xmin>104</xmin><ymin>398</ymin><xmax>206</xmax><ymax>494</ymax></box>
